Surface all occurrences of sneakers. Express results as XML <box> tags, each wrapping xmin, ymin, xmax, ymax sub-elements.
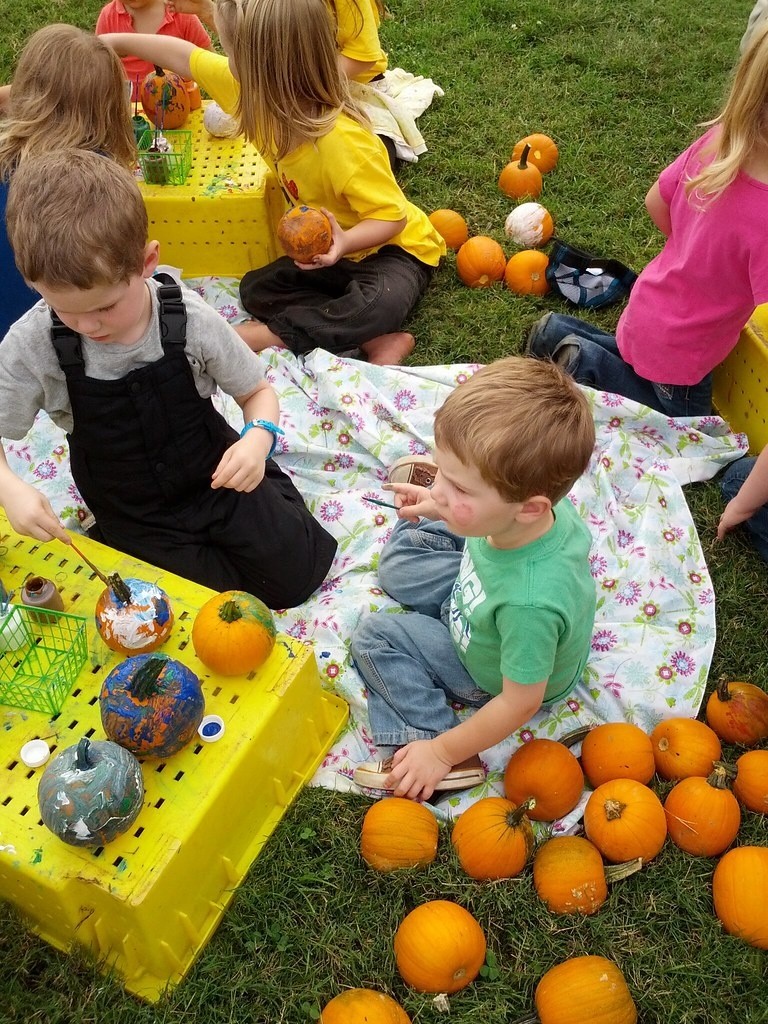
<box><xmin>382</xmin><ymin>455</ymin><xmax>443</xmax><ymax>495</ymax></box>
<box><xmin>346</xmin><ymin>741</ymin><xmax>489</xmax><ymax>794</ymax></box>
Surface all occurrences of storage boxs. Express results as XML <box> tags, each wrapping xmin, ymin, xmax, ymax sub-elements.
<box><xmin>129</xmin><ymin>94</ymin><xmax>286</xmax><ymax>282</ymax></box>
<box><xmin>715</xmin><ymin>303</ymin><xmax>768</xmax><ymax>455</ymax></box>
<box><xmin>0</xmin><ymin>506</ymin><xmax>351</xmax><ymax>1003</ymax></box>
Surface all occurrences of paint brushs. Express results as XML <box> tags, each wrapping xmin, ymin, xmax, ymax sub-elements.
<box><xmin>155</xmin><ymin>100</ymin><xmax>158</xmax><ymax>151</ymax></box>
<box><xmin>135</xmin><ymin>73</ymin><xmax>139</xmax><ymax>121</ymax></box>
<box><xmin>69</xmin><ymin>542</ymin><xmax>119</xmax><ymax>602</ymax></box>
<box><xmin>159</xmin><ymin>93</ymin><xmax>165</xmax><ymax>142</ymax></box>
<box><xmin>362</xmin><ymin>496</ymin><xmax>401</xmax><ymax>510</ymax></box>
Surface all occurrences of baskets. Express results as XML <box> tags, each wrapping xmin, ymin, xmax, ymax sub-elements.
<box><xmin>0</xmin><ymin>599</ymin><xmax>88</xmax><ymax>717</ymax></box>
<box><xmin>134</xmin><ymin>127</ymin><xmax>195</xmax><ymax>186</ymax></box>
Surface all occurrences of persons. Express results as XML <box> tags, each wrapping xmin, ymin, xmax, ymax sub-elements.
<box><xmin>521</xmin><ymin>2</ymin><xmax>767</xmax><ymax>418</ymax></box>
<box><xmin>326</xmin><ymin>0</ymin><xmax>397</xmax><ymax>167</ymax></box>
<box><xmin>716</xmin><ymin>439</ymin><xmax>768</xmax><ymax>555</ymax></box>
<box><xmin>0</xmin><ymin>148</ymin><xmax>340</xmax><ymax>610</ymax></box>
<box><xmin>0</xmin><ymin>24</ymin><xmax>138</xmax><ymax>343</ymax></box>
<box><xmin>352</xmin><ymin>357</ymin><xmax>597</xmax><ymax>790</ymax></box>
<box><xmin>94</xmin><ymin>0</ymin><xmax>445</xmax><ymax>365</ymax></box>
<box><xmin>94</xmin><ymin>0</ymin><xmax>219</xmax><ymax>104</ymax></box>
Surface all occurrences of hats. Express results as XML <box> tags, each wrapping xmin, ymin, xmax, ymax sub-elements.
<box><xmin>541</xmin><ymin>232</ymin><xmax>644</xmax><ymax>314</ymax></box>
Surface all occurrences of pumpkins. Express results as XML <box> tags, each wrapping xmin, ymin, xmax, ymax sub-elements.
<box><xmin>504</xmin><ymin>202</ymin><xmax>553</xmax><ymax>249</ymax></box>
<box><xmin>278</xmin><ymin>205</ymin><xmax>331</xmax><ymax>263</ymax></box>
<box><xmin>36</xmin><ymin>573</ymin><xmax>278</xmax><ymax>847</ymax></box>
<box><xmin>204</xmin><ymin>103</ymin><xmax>239</xmax><ymax>137</ymax></box>
<box><xmin>428</xmin><ymin>209</ymin><xmax>469</xmax><ymax>249</ymax></box>
<box><xmin>316</xmin><ymin>674</ymin><xmax>768</xmax><ymax>1024</ymax></box>
<box><xmin>458</xmin><ymin>236</ymin><xmax>506</xmax><ymax>288</ymax></box>
<box><xmin>503</xmin><ymin>250</ymin><xmax>551</xmax><ymax>296</ymax></box>
<box><xmin>141</xmin><ymin>63</ymin><xmax>190</xmax><ymax>130</ymax></box>
<box><xmin>512</xmin><ymin>133</ymin><xmax>558</xmax><ymax>173</ymax></box>
<box><xmin>498</xmin><ymin>143</ymin><xmax>543</xmax><ymax>201</ymax></box>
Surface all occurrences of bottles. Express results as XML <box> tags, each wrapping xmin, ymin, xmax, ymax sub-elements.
<box><xmin>0</xmin><ymin>603</ymin><xmax>28</xmax><ymax>653</ymax></box>
<box><xmin>144</xmin><ymin>148</ymin><xmax>170</xmax><ymax>184</ymax></box>
<box><xmin>131</xmin><ymin>115</ymin><xmax>153</xmax><ymax>151</ymax></box>
<box><xmin>150</xmin><ymin>137</ymin><xmax>172</xmax><ymax>168</ymax></box>
<box><xmin>182</xmin><ymin>77</ymin><xmax>202</xmax><ymax>112</ymax></box>
<box><xmin>21</xmin><ymin>576</ymin><xmax>66</xmax><ymax>624</ymax></box>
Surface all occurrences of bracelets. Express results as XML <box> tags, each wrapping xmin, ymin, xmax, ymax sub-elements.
<box><xmin>241</xmin><ymin>420</ymin><xmax>285</xmax><ymax>460</ymax></box>
